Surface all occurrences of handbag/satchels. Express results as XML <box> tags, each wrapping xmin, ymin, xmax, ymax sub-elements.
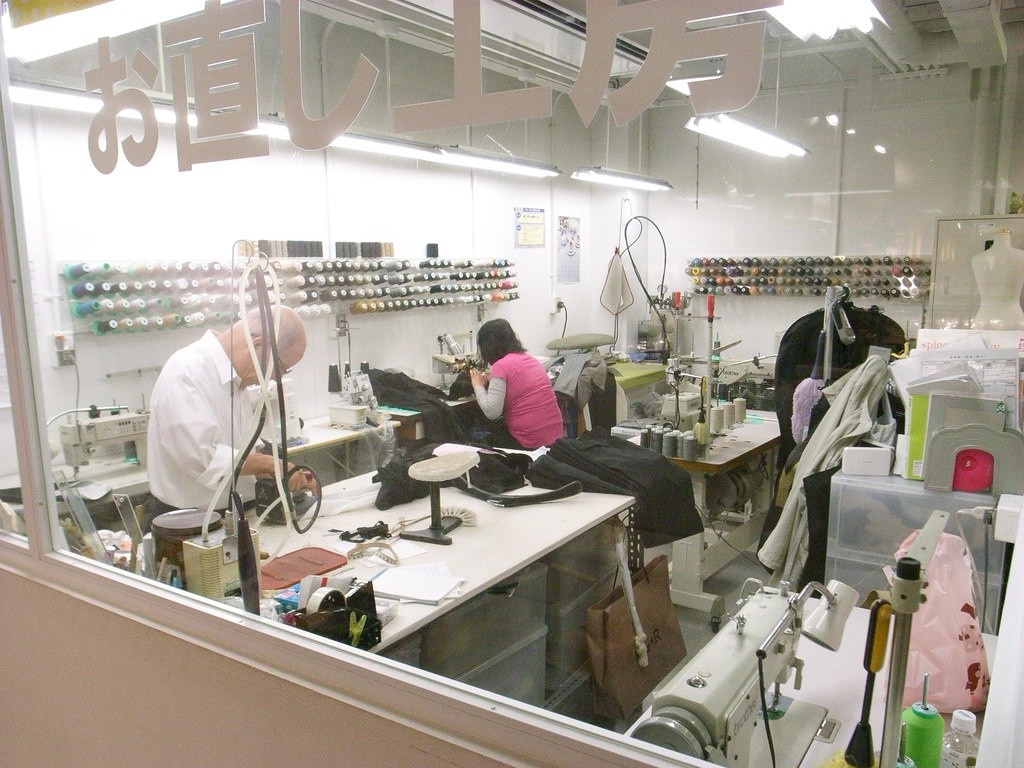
<box><xmin>585</xmin><ymin>551</ymin><xmax>687</xmax><ymax>720</ymax></box>
<box><xmin>882</xmin><ymin>529</ymin><xmax>991</xmax><ymax>712</ymax></box>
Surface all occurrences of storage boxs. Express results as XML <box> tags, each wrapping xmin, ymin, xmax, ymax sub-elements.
<box><xmin>376</xmin><ymin>514</ymin><xmax>628</xmax><ymax>709</ymax></box>
<box><xmin>823</xmin><ymin>467</ymin><xmax>1006</xmax><ymax>634</ymax></box>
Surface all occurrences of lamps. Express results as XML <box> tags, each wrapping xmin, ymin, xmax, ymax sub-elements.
<box><xmin>3</xmin><ymin>0</ymin><xmax>893</xmax><ymax>192</ymax></box>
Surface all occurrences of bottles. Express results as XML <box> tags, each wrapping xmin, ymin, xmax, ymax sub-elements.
<box><xmin>942</xmin><ymin>708</ymin><xmax>979</xmax><ymax>768</ymax></box>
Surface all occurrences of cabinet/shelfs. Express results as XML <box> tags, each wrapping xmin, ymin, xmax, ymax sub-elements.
<box><xmin>924</xmin><ymin>213</ymin><xmax>1024</xmax><ymax>332</ymax></box>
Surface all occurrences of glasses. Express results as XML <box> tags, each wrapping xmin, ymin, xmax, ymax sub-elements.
<box><xmin>250</xmin><ymin>333</ymin><xmax>294</xmax><ymax>377</ymax></box>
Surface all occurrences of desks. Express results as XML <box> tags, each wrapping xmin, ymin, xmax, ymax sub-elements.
<box><xmin>143</xmin><ymin>468</ymin><xmax>637</xmax><ymax>653</ymax></box>
<box><xmin>0</xmin><ymin>416</ymin><xmax>396</xmax><ymax>530</ymax></box>
<box><xmin>626</xmin><ymin>409</ymin><xmax>784</xmax><ymax>616</ymax></box>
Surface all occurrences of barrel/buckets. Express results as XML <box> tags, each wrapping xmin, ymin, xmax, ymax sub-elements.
<box><xmin>151</xmin><ymin>506</ymin><xmax>221</xmax><ymax>593</ymax></box>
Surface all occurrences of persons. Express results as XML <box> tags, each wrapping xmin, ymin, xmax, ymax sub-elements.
<box><xmin>471</xmin><ymin>321</ymin><xmax>566</xmax><ymax>449</ymax></box>
<box><xmin>148</xmin><ymin>304</ymin><xmax>313</xmax><ymax>530</ymax></box>
<box><xmin>757</xmin><ymin>304</ymin><xmax>906</xmax><ymax>575</ymax></box>
